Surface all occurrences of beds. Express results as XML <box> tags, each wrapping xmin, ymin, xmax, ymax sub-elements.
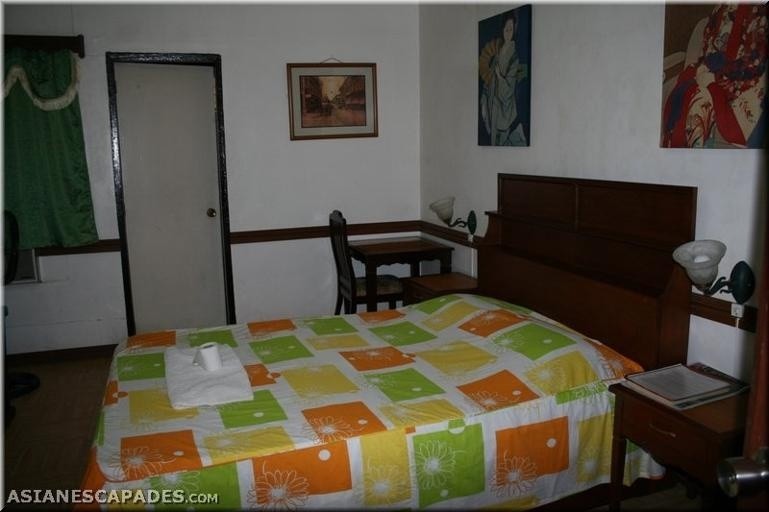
<box><xmin>79</xmin><ymin>173</ymin><xmax>697</xmax><ymax>511</ymax></box>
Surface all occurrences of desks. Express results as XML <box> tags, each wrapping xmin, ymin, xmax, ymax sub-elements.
<box><xmin>349</xmin><ymin>237</ymin><xmax>455</xmax><ymax>313</ymax></box>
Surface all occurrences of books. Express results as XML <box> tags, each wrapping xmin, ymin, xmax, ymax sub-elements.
<box><xmin>620</xmin><ymin>362</ymin><xmax>750</xmax><ymax>412</ymax></box>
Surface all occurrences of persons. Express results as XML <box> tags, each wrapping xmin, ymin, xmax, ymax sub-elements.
<box><xmin>480</xmin><ymin>10</ymin><xmax>527</xmax><ymax>145</ymax></box>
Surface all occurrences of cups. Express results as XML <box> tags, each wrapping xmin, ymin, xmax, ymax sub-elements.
<box><xmin>197</xmin><ymin>341</ymin><xmax>222</xmax><ymax>372</ymax></box>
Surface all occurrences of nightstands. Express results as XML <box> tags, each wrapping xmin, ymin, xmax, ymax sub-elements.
<box><xmin>608</xmin><ymin>365</ymin><xmax>752</xmax><ymax>507</ymax></box>
<box><xmin>402</xmin><ymin>272</ymin><xmax>479</xmax><ymax>306</ymax></box>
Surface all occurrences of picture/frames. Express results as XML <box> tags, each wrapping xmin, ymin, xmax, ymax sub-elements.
<box><xmin>286</xmin><ymin>62</ymin><xmax>379</xmax><ymax>140</ymax></box>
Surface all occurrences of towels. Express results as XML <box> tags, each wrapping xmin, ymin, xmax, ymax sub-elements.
<box><xmin>164</xmin><ymin>341</ymin><xmax>256</xmax><ymax>411</ymax></box>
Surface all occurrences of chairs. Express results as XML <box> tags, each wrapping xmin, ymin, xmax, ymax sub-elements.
<box><xmin>328</xmin><ymin>210</ymin><xmax>403</xmax><ymax>314</ymax></box>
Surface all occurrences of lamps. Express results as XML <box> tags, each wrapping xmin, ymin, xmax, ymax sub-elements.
<box><xmin>430</xmin><ymin>195</ymin><xmax>477</xmax><ymax>235</ymax></box>
<box><xmin>671</xmin><ymin>237</ymin><xmax>757</xmax><ymax>306</ymax></box>
